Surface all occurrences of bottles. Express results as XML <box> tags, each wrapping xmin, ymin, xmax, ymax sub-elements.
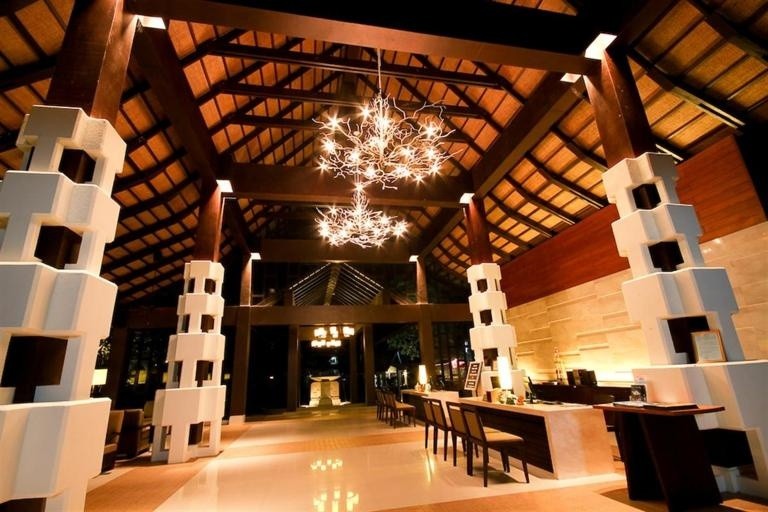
<box><xmin>555</xmin><ymin>368</ymin><xmax>566</xmax><ymax>385</ymax></box>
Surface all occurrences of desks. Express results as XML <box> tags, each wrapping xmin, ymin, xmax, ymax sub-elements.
<box><xmin>458</xmin><ymin>396</ymin><xmax>615</xmax><ymax>483</ymax></box>
<box><xmin>592</xmin><ymin>399</ymin><xmax>726</xmax><ymax>512</ymax></box>
<box><xmin>528</xmin><ymin>381</ymin><xmax>647</xmax><ymax>432</ymax></box>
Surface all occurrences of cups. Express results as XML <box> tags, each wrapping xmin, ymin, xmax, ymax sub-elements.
<box><xmin>414</xmin><ymin>375</ymin><xmax>448</xmax><ymax>392</ymax></box>
<box><xmin>498</xmin><ymin>390</ymin><xmax>538</xmax><ymax>405</ymax></box>
<box><xmin>630</xmin><ymin>386</ymin><xmax>641</xmax><ymax>402</ymax></box>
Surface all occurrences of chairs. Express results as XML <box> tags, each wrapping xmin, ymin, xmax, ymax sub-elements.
<box><xmin>375</xmin><ymin>388</ymin><xmax>530</xmax><ymax>487</ymax></box>
<box><xmin>100</xmin><ymin>399</ymin><xmax>154</xmax><ymax>472</ymax></box>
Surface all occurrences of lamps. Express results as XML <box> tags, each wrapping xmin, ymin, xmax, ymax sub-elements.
<box><xmin>419</xmin><ymin>365</ymin><xmax>427</xmax><ymax>392</ymax></box>
<box><xmin>312</xmin><ymin>48</ymin><xmax>463</xmax><ymax>192</ymax></box>
<box><xmin>497</xmin><ymin>357</ymin><xmax>512</xmax><ymax>403</ymax></box>
<box><xmin>312</xmin><ymin>130</ymin><xmax>408</xmax><ymax>248</ymax></box>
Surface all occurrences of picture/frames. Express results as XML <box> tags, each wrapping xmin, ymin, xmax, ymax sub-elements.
<box><xmin>691</xmin><ymin>330</ymin><xmax>727</xmax><ymax>363</ymax></box>
